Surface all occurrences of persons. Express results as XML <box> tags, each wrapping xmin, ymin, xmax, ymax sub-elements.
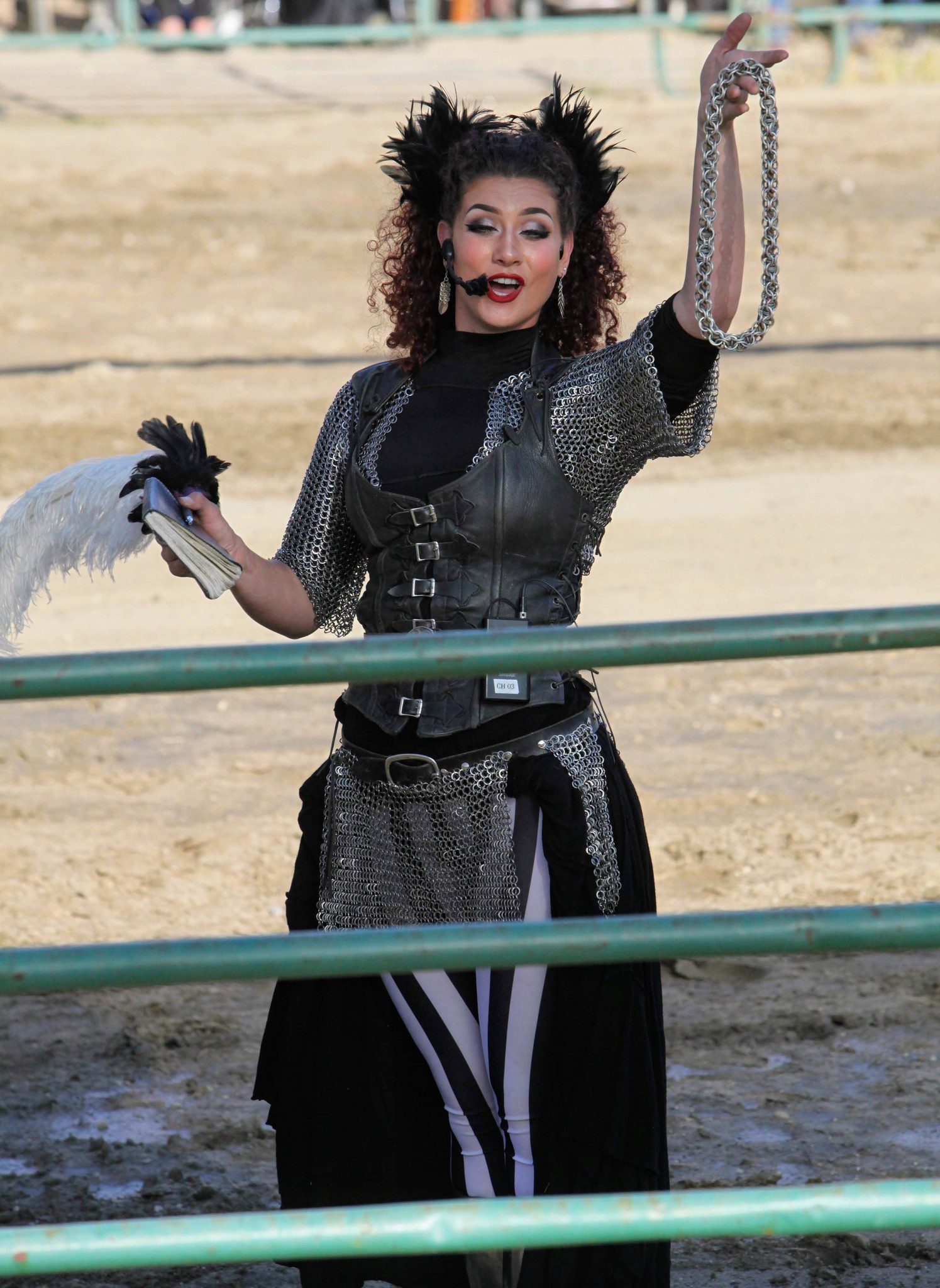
<box><xmin>158</xmin><ymin>11</ymin><xmax>791</xmax><ymax>1288</ymax></box>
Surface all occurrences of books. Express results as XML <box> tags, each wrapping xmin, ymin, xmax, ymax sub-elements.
<box><xmin>141</xmin><ymin>477</ymin><xmax>242</xmax><ymax>600</ymax></box>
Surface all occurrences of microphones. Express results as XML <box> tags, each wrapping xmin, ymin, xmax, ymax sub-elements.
<box><xmin>446</xmin><ymin>258</ymin><xmax>489</xmax><ymax>296</ymax></box>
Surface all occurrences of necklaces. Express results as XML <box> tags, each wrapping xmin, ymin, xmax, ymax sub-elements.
<box><xmin>693</xmin><ymin>58</ymin><xmax>779</xmax><ymax>354</ymax></box>
